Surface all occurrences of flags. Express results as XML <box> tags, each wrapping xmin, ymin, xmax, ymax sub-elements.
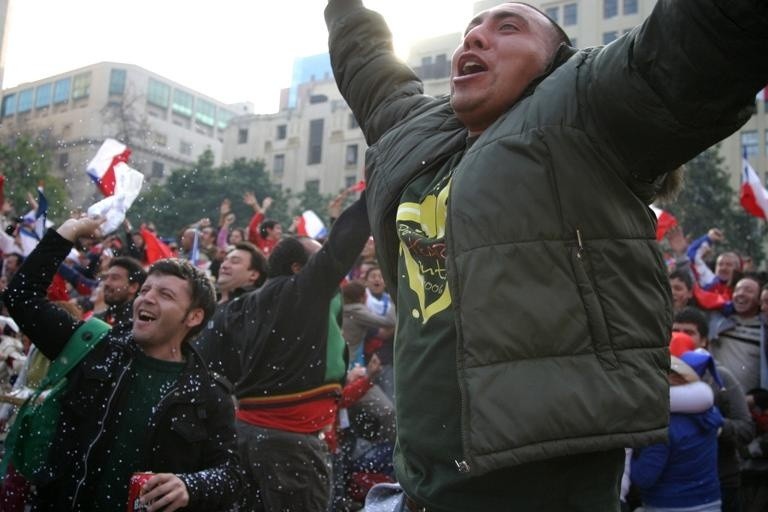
<box><xmin>741</xmin><ymin>145</ymin><xmax>768</xmax><ymax>222</ymax></box>
<box><xmin>85</xmin><ymin>139</ymin><xmax>131</xmax><ymax>196</ymax></box>
<box><xmin>755</xmin><ymin>88</ymin><xmax>768</xmax><ymax>103</ymax></box>
<box><xmin>87</xmin><ymin>163</ymin><xmax>146</xmax><ymax>237</ymax></box>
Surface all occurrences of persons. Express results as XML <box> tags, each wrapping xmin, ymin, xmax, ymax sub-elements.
<box><xmin>322</xmin><ymin>0</ymin><xmax>768</xmax><ymax>512</ymax></box>
<box><xmin>0</xmin><ymin>181</ymin><xmax>768</xmax><ymax>512</ymax></box>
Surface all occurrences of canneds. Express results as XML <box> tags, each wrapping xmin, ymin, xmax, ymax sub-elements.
<box><xmin>128</xmin><ymin>471</ymin><xmax>161</xmax><ymax>512</ymax></box>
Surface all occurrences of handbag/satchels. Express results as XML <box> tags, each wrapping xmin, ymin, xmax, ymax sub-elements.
<box><xmin>1</xmin><ymin>379</ymin><xmax>64</xmax><ymax>483</ymax></box>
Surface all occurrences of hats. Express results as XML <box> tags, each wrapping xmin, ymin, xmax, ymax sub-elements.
<box><xmin>670</xmin><ymin>348</ymin><xmax>725</xmax><ymax>391</ymax></box>
